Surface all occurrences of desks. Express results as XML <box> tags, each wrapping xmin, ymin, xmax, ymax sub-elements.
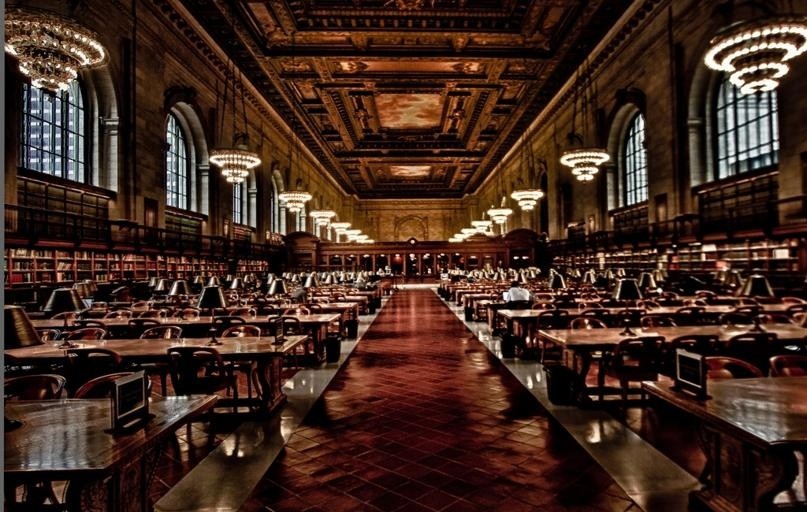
<box><xmin>332</xmin><ymin>287</ymin><xmax>375</xmax><ymax>314</ymax></box>
<box><xmin>4</xmin><ymin>396</ymin><xmax>220</xmax><ymax>511</ymax></box>
<box><xmin>231</xmin><ymin>310</ymin><xmax>343</xmax><ymax>363</ymax></box>
<box><xmin>304</xmin><ymin>302</ymin><xmax>360</xmax><ymax>336</ymax></box>
<box><xmin>328</xmin><ymin>295</ymin><xmax>369</xmax><ymax>316</ymax></box>
<box><xmin>639</xmin><ymin>379</ymin><xmax>807</xmax><ymax>512</ymax></box>
<box><xmin>438</xmin><ymin>280</ymin><xmax>488</xmax><ymax>322</ymax></box>
<box><xmin>538</xmin><ymin>318</ymin><xmax>806</xmax><ymax>402</ymax></box>
<box><xmin>5</xmin><ymin>333</ymin><xmax>309</xmax><ymax>421</ymax></box>
<box><xmin>352</xmin><ymin>281</ymin><xmax>392</xmax><ymax>295</ymax></box>
<box><xmin>489</xmin><ymin>305</ymin><xmax>749</xmax><ymax>359</ymax></box>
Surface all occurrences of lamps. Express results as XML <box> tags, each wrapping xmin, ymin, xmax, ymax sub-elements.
<box><xmin>356</xmin><ymin>239</ymin><xmax>377</xmax><ymax>245</ymax></box>
<box><xmin>485</xmin><ymin>159</ymin><xmax>514</xmax><ymax>226</ymax></box>
<box><xmin>2</xmin><ymin>2</ymin><xmax>113</xmax><ymax>103</ymax></box>
<box><xmin>291</xmin><ymin>274</ymin><xmax>302</xmax><ymax>283</ymax></box>
<box><xmin>207</xmin><ymin>276</ymin><xmax>222</xmax><ymax>287</ymax></box>
<box><xmin>300</xmin><ymin>272</ymin><xmax>307</xmax><ymax>278</ymax></box>
<box><xmin>556</xmin><ymin>42</ymin><xmax>611</xmax><ymax>185</ymax></box>
<box><xmin>571</xmin><ymin>267</ymin><xmax>581</xmax><ymax>279</ymax></box>
<box><xmin>470</xmin><ymin>220</ymin><xmax>493</xmax><ymax>235</ymax></box>
<box><xmin>307</xmin><ymin>208</ymin><xmax>337</xmax><ymax>229</ymax></box>
<box><xmin>209</xmin><ymin>15</ymin><xmax>263</xmax><ymax>187</ymax></box>
<box><xmin>266</xmin><ymin>278</ymin><xmax>289</xmax><ymax>306</ymax></box>
<box><xmin>4</xmin><ymin>304</ymin><xmax>46</xmax><ymax>434</ymax></box>
<box><xmin>340</xmin><ymin>273</ymin><xmax>351</xmax><ymax>282</ymax></box>
<box><xmin>167</xmin><ymin>280</ymin><xmax>194</xmax><ymax>310</ymax></box>
<box><xmin>602</xmin><ymin>268</ymin><xmax>614</xmax><ymax>280</ymax></box>
<box><xmin>616</xmin><ymin>267</ymin><xmax>628</xmax><ymax>277</ymax></box>
<box><xmin>612</xmin><ymin>277</ymin><xmax>645</xmax><ymax>335</ymax></box>
<box><xmin>452</xmin><ymin>233</ymin><xmax>466</xmax><ymax>241</ymax></box>
<box><xmin>277</xmin><ymin>55</ymin><xmax>313</xmax><ymax>216</ymax></box>
<box><xmin>508</xmin><ymin>127</ymin><xmax>546</xmax><ymax>213</ymax></box>
<box><xmin>637</xmin><ymin>272</ymin><xmax>658</xmax><ymax>302</ymax></box>
<box><xmin>492</xmin><ymin>272</ymin><xmax>505</xmax><ymax>281</ymax></box>
<box><xmin>267</xmin><ymin>273</ymin><xmax>277</xmax><ymax>284</ymax></box>
<box><xmin>321</xmin><ymin>272</ymin><xmax>327</xmax><ymax>280</ymax></box>
<box><xmin>285</xmin><ymin>272</ymin><xmax>292</xmax><ymax>279</ymax></box>
<box><xmin>193</xmin><ymin>275</ymin><xmax>207</xmax><ymax>286</ymax></box>
<box><xmin>230</xmin><ymin>277</ymin><xmax>246</xmax><ymax>307</ymax></box>
<box><xmin>196</xmin><ymin>286</ymin><xmax>227</xmax><ymax>346</ymax></box>
<box><xmin>515</xmin><ymin>268</ymin><xmax>528</xmax><ymax>285</ymax></box>
<box><xmin>353</xmin><ymin>234</ymin><xmax>371</xmax><ymax>241</ymax></box>
<box><xmin>328</xmin><ymin>222</ymin><xmax>352</xmax><ymax>234</ymax></box>
<box><xmin>148</xmin><ymin>275</ymin><xmax>158</xmax><ymax>287</ymax></box>
<box><xmin>44</xmin><ymin>288</ymin><xmax>87</xmax><ymax>350</ymax></box>
<box><xmin>446</xmin><ymin>237</ymin><xmax>462</xmax><ymax>244</ymax></box>
<box><xmin>72</xmin><ymin>283</ymin><xmax>95</xmax><ymax>300</ymax></box>
<box><xmin>324</xmin><ymin>273</ymin><xmax>339</xmax><ymax>284</ymax></box>
<box><xmin>581</xmin><ymin>272</ymin><xmax>597</xmax><ymax>286</ymax></box>
<box><xmin>342</xmin><ymin>230</ymin><xmax>361</xmax><ymax>239</ymax></box>
<box><xmin>244</xmin><ymin>274</ymin><xmax>250</xmax><ymax>284</ymax></box>
<box><xmin>461</xmin><ymin>227</ymin><xmax>477</xmax><ymax>237</ymax></box>
<box><xmin>737</xmin><ymin>273</ymin><xmax>777</xmax><ymax>334</ymax></box>
<box><xmin>547</xmin><ymin>274</ymin><xmax>567</xmax><ymax>296</ymax></box>
<box><xmin>83</xmin><ymin>278</ymin><xmax>99</xmax><ymax>293</ymax></box>
<box><xmin>225</xmin><ymin>274</ymin><xmax>233</xmax><ymax>283</ymax></box>
<box><xmin>652</xmin><ymin>269</ymin><xmax>665</xmax><ymax>284</ymax></box>
<box><xmin>526</xmin><ymin>266</ymin><xmax>537</xmax><ymax>279</ymax></box>
<box><xmin>701</xmin><ymin>2</ymin><xmax>807</xmax><ymax>98</ymax></box>
<box><xmin>303</xmin><ymin>271</ymin><xmax>319</xmax><ymax>288</ymax></box>
<box><xmin>153</xmin><ymin>278</ymin><xmax>171</xmax><ymax>293</ymax></box>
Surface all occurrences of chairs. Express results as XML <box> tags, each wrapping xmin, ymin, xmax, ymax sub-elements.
<box><xmin>229</xmin><ymin>307</ymin><xmax>257</xmax><ymax>317</ymax></box>
<box><xmin>719</xmin><ymin>305</ymin><xmax>766</xmax><ymax>326</ymax></box>
<box><xmin>535</xmin><ymin>310</ymin><xmax>570</xmax><ymax>365</ymax></box>
<box><xmin>598</xmin><ymin>336</ymin><xmax>666</xmax><ymax>402</ymax></box>
<box><xmin>72</xmin><ymin>371</ymin><xmax>166</xmax><ymax>511</ymax></box>
<box><xmin>166</xmin><ymin>346</ymin><xmax>239</xmax><ymax>440</ymax></box>
<box><xmin>170</xmin><ymin>308</ymin><xmax>200</xmax><ymax>319</ymax></box>
<box><xmin>103</xmin><ymin>309</ymin><xmax>133</xmax><ymax>320</ymax></box>
<box><xmin>66</xmin><ymin>328</ymin><xmax>107</xmax><ymax>343</ymax></box>
<box><xmin>49</xmin><ymin>311</ymin><xmax>83</xmax><ymax>322</ymax></box>
<box><xmin>4</xmin><ymin>374</ymin><xmax>67</xmax><ymax>403</ymax></box>
<box><xmin>299</xmin><ymin>302</ymin><xmax>322</xmax><ymax>314</ymax></box>
<box><xmin>209</xmin><ymin>316</ymin><xmax>247</xmax><ymax>340</ymax></box>
<box><xmin>268</xmin><ymin>316</ymin><xmax>310</xmax><ymax>372</ymax></box>
<box><xmin>639</xmin><ymin>316</ymin><xmax>678</xmax><ymax>327</ymax></box>
<box><xmin>61</xmin><ymin>347</ymin><xmax>124</xmax><ymax>371</ymax></box>
<box><xmin>570</xmin><ymin>316</ymin><xmax>608</xmax><ymax>329</ymax></box>
<box><xmin>580</xmin><ymin>309</ymin><xmax>610</xmax><ymax>320</ymax></box>
<box><xmin>768</xmin><ymin>349</ymin><xmax>807</xmax><ymax>378</ymax></box>
<box><xmin>128</xmin><ymin>318</ymin><xmax>161</xmax><ymax>336</ymax></box>
<box><xmin>613</xmin><ymin>309</ymin><xmax>643</xmax><ymax>324</ymax></box>
<box><xmin>137</xmin><ymin>324</ymin><xmax>179</xmax><ymax>397</ymax></box>
<box><xmin>674</xmin><ymin>306</ymin><xmax>706</xmax><ymax>324</ymax></box>
<box><xmin>490</xmin><ymin>292</ymin><xmax>503</xmax><ymax>300</ymax></box>
<box><xmin>68</xmin><ymin>320</ymin><xmax>113</xmax><ymax>341</ymax></box>
<box><xmin>137</xmin><ymin>309</ymin><xmax>166</xmax><ymax>320</ymax></box>
<box><xmin>35</xmin><ymin>328</ymin><xmax>62</xmax><ymax>347</ymax></box>
<box><xmin>671</xmin><ymin>334</ymin><xmax>719</xmax><ymax>356</ymax></box>
<box><xmin>281</xmin><ymin>307</ymin><xmax>310</xmax><ymax>317</ymax></box>
<box><xmin>220</xmin><ymin>325</ymin><xmax>261</xmax><ymax>400</ymax></box>
<box><xmin>704</xmin><ymin>354</ymin><xmax>765</xmax><ymax>379</ymax></box>
<box><xmin>328</xmin><ymin>292</ymin><xmax>345</xmax><ymax>304</ymax></box>
<box><xmin>727</xmin><ymin>333</ymin><xmax>767</xmax><ymax>354</ymax></box>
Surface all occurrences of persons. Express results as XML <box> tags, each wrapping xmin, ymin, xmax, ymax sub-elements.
<box><xmin>652</xmin><ymin>263</ymin><xmax>668</xmax><ymax>290</ymax></box>
<box><xmin>482</xmin><ymin>263</ymin><xmax>494</xmax><ymax>276</ymax></box>
<box><xmin>505</xmin><ymin>280</ymin><xmax>531</xmax><ymax>344</ymax></box>
